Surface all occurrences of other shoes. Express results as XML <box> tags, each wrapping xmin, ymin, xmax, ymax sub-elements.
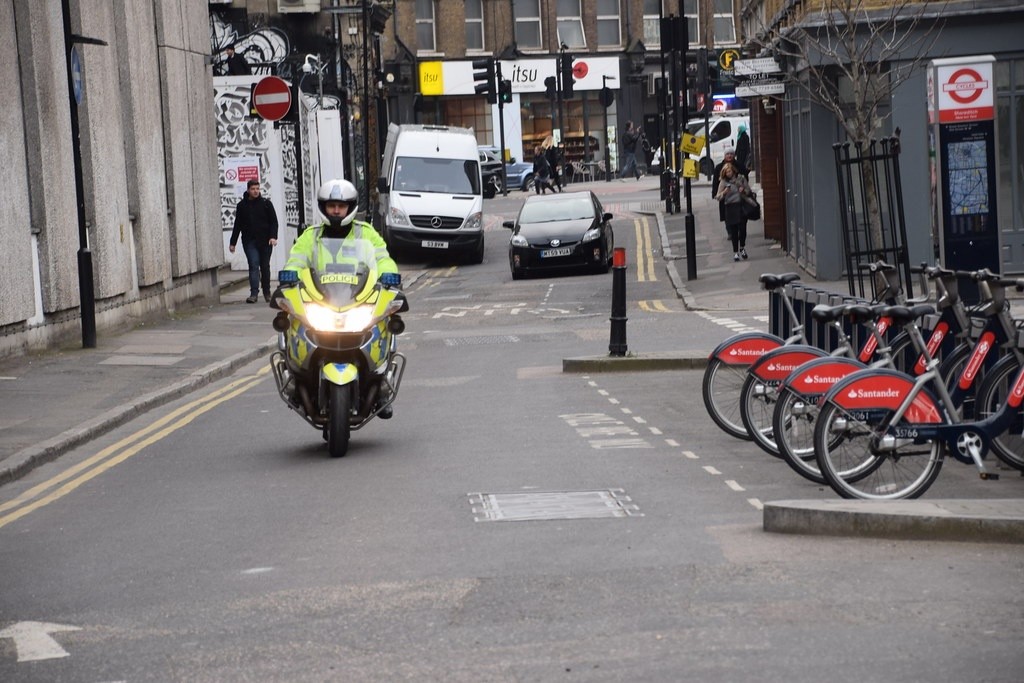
<box><xmin>727</xmin><ymin>234</ymin><xmax>732</xmax><ymax>240</ymax></box>
<box><xmin>264</xmin><ymin>293</ymin><xmax>271</xmax><ymax>302</ymax></box>
<box><xmin>734</xmin><ymin>253</ymin><xmax>739</xmax><ymax>260</ymax></box>
<box><xmin>741</xmin><ymin>250</ymin><xmax>748</xmax><ymax>259</ymax></box>
<box><xmin>246</xmin><ymin>290</ymin><xmax>259</xmax><ymax>303</ymax></box>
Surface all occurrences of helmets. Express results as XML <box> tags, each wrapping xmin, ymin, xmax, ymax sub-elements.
<box><xmin>316</xmin><ymin>179</ymin><xmax>360</xmax><ymax>226</ymax></box>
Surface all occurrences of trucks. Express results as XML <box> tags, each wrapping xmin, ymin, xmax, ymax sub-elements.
<box><xmin>376</xmin><ymin>122</ymin><xmax>495</xmax><ymax>264</ymax></box>
<box><xmin>652</xmin><ymin>113</ymin><xmax>750</xmax><ymax>175</ymax></box>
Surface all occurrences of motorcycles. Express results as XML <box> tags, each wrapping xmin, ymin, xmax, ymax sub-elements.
<box><xmin>269</xmin><ymin>238</ymin><xmax>409</xmax><ymax>456</ymax></box>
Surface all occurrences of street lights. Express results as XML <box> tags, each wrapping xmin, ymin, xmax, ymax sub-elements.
<box><xmin>67</xmin><ymin>32</ymin><xmax>109</xmax><ymax>348</ymax></box>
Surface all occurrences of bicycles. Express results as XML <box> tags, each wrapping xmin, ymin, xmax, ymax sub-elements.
<box><xmin>702</xmin><ymin>261</ymin><xmax>1024</xmax><ymax>499</ymax></box>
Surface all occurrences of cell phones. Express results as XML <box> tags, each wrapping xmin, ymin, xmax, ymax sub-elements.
<box><xmin>727</xmin><ymin>185</ymin><xmax>730</xmax><ymax>188</ymax></box>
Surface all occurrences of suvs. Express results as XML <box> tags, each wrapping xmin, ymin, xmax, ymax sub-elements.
<box><xmin>479</xmin><ymin>145</ymin><xmax>537</xmax><ymax>197</ymax></box>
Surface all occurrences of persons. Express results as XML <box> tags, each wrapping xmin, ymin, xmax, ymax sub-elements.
<box><xmin>229</xmin><ymin>181</ymin><xmax>279</xmax><ymax>304</ymax></box>
<box><xmin>620</xmin><ymin>120</ymin><xmax>644</xmax><ymax>184</ymax></box>
<box><xmin>532</xmin><ymin>146</ymin><xmax>557</xmax><ymax>195</ymax></box>
<box><xmin>712</xmin><ymin>147</ymin><xmax>750</xmax><ymax>240</ymax></box>
<box><xmin>542</xmin><ymin>135</ymin><xmax>563</xmax><ymax>192</ymax></box>
<box><xmin>714</xmin><ymin>162</ymin><xmax>754</xmax><ymax>262</ymax></box>
<box><xmin>274</xmin><ymin>180</ymin><xmax>403</xmax><ymax>418</ymax></box>
<box><xmin>736</xmin><ymin>126</ymin><xmax>750</xmax><ymax>172</ymax></box>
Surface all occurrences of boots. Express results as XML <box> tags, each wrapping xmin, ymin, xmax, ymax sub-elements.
<box><xmin>287</xmin><ymin>397</ymin><xmax>299</xmax><ymax>409</ymax></box>
<box><xmin>375</xmin><ymin>373</ymin><xmax>393</xmax><ymax>419</ymax></box>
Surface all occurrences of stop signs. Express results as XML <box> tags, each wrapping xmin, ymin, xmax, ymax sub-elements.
<box><xmin>253</xmin><ymin>76</ymin><xmax>292</xmax><ymax>122</ymax></box>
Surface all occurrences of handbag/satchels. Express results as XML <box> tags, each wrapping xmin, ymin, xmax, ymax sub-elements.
<box><xmin>742</xmin><ymin>192</ymin><xmax>760</xmax><ymax>220</ymax></box>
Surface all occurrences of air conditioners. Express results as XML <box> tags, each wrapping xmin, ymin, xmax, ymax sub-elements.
<box><xmin>647</xmin><ymin>73</ymin><xmax>672</xmax><ymax>95</ymax></box>
<box><xmin>277</xmin><ymin>0</ymin><xmax>321</xmax><ymax>13</ymax></box>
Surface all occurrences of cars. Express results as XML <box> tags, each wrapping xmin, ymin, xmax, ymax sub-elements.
<box><xmin>502</xmin><ymin>190</ymin><xmax>615</xmax><ymax>281</ymax></box>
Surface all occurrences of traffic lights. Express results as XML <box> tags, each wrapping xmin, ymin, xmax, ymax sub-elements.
<box><xmin>545</xmin><ymin>78</ymin><xmax>557</xmax><ymax>101</ymax></box>
<box><xmin>558</xmin><ymin>57</ymin><xmax>576</xmax><ymax>100</ymax></box>
<box><xmin>500</xmin><ymin>79</ymin><xmax>514</xmax><ymax>104</ymax></box>
<box><xmin>472</xmin><ymin>58</ymin><xmax>496</xmax><ymax>103</ymax></box>
